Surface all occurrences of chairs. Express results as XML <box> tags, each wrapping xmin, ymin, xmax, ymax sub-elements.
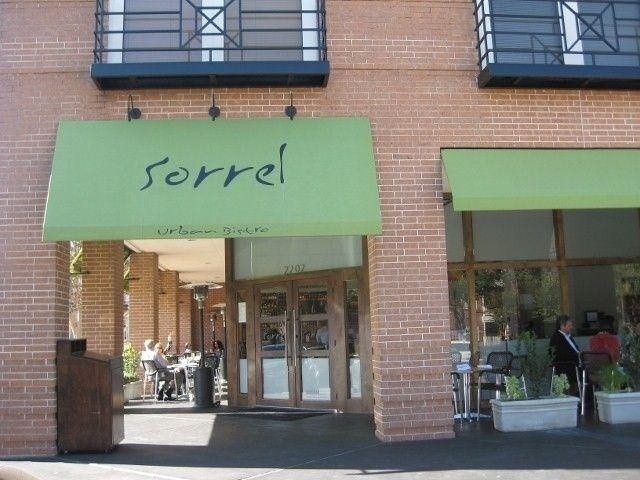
<box><xmin>450</xmin><ymin>351</ymin><xmax>528</xmax><ymax>424</ymax></box>
<box><xmin>142</xmin><ymin>352</ymin><xmax>221</xmax><ymax>403</ymax></box>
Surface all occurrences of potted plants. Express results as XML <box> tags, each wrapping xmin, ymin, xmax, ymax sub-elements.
<box><xmin>488</xmin><ymin>328</ymin><xmax>640</xmax><ymax>433</ymax></box>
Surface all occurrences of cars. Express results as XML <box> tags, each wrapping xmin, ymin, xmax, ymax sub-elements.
<box><xmin>452</xmin><ymin>340</ymin><xmax>476</xmax><ymax>364</ymax></box>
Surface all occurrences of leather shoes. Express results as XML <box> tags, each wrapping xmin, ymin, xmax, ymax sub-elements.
<box><xmin>164</xmin><ymin>397</ymin><xmax>177</xmax><ymax>401</ymax></box>
<box><xmin>157</xmin><ymin>396</ymin><xmax>161</xmax><ymax>400</ymax></box>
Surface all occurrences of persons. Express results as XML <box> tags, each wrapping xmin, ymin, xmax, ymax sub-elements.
<box><xmin>154</xmin><ymin>343</ymin><xmax>183</xmax><ymax>396</ymax></box>
<box><xmin>546</xmin><ymin>315</ymin><xmax>593</xmax><ymax>421</ymax></box>
<box><xmin>140</xmin><ymin>339</ymin><xmax>176</xmax><ymax>401</ymax></box>
<box><xmin>315</xmin><ymin>321</ymin><xmax>329</xmax><ymax>351</ymax></box>
<box><xmin>212</xmin><ymin>340</ymin><xmax>224</xmax><ymax>380</ymax></box>
<box><xmin>589</xmin><ymin>315</ymin><xmax>623</xmax><ymax>372</ymax></box>
